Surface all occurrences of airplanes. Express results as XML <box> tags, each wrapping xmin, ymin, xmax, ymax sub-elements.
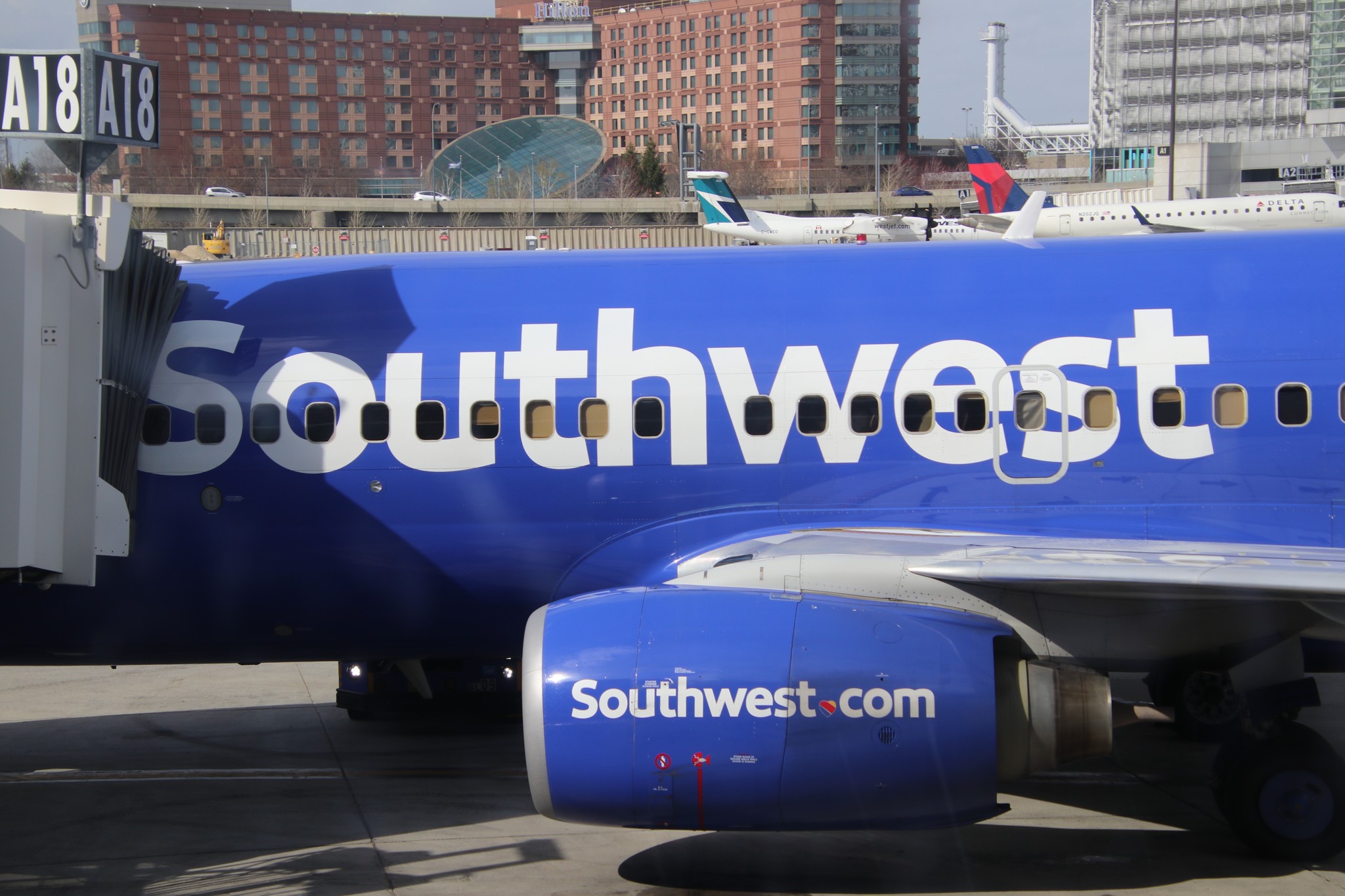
<box><xmin>685</xmin><ymin>171</ymin><xmax>1345</xmax><ymax>251</ymax></box>
<box><xmin>0</xmin><ymin>49</ymin><xmax>1345</xmax><ymax>896</ymax></box>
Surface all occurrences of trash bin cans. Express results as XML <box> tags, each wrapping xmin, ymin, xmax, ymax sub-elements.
<box><xmin>526</xmin><ymin>236</ymin><xmax>538</xmax><ymax>250</ymax></box>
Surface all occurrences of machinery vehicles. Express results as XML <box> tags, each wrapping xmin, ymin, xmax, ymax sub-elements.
<box><xmin>201</xmin><ymin>219</ymin><xmax>231</xmax><ymax>256</ymax></box>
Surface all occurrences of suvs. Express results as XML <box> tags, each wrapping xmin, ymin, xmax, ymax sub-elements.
<box><xmin>205</xmin><ymin>187</ymin><xmax>247</xmax><ymax>197</ymax></box>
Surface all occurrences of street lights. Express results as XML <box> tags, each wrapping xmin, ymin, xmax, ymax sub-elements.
<box><xmin>431</xmin><ymin>102</ymin><xmax>441</xmax><ymax>202</ymax></box>
<box><xmin>259</xmin><ymin>156</ymin><xmax>269</xmax><ymax>227</ymax></box>
<box><xmin>531</xmin><ymin>152</ymin><xmax>536</xmax><ymax>227</ymax></box>
<box><xmin>962</xmin><ymin>107</ymin><xmax>973</xmax><ymax>138</ymax></box>
<box><xmin>877</xmin><ymin>142</ymin><xmax>883</xmax><ymax>217</ymax></box>
<box><xmin>797</xmin><ymin>98</ymin><xmax>813</xmax><ymax>199</ymax></box>
<box><xmin>1014</xmin><ymin>164</ymin><xmax>1031</xmax><ymax>195</ymax></box>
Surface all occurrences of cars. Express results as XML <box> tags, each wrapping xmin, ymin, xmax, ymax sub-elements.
<box><xmin>413</xmin><ymin>190</ymin><xmax>455</xmax><ymax>201</ymax></box>
<box><xmin>891</xmin><ymin>186</ymin><xmax>934</xmax><ymax>196</ymax></box>
